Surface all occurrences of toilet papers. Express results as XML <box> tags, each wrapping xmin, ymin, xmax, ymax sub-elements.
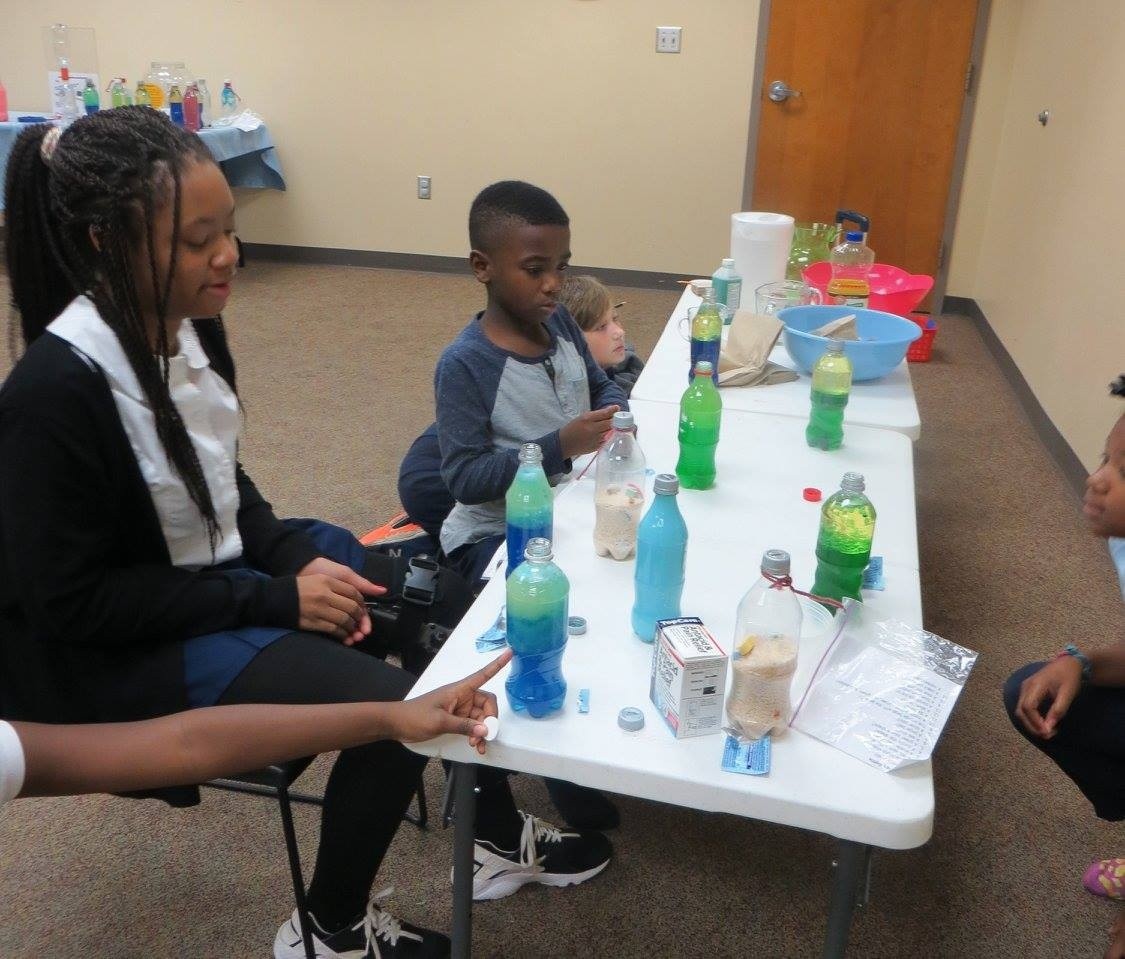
<box><xmin>729</xmin><ymin>211</ymin><xmax>795</xmax><ymax>306</ymax></box>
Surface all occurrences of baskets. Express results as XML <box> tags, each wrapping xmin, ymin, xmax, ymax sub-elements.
<box><xmin>905</xmin><ymin>314</ymin><xmax>938</xmax><ymax>362</ymax></box>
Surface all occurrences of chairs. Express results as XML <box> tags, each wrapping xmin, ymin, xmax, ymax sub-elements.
<box><xmin>203</xmin><ymin>748</ymin><xmax>429</xmax><ymax>959</ymax></box>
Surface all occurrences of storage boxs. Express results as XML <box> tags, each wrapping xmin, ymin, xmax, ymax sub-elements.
<box><xmin>906</xmin><ymin>315</ymin><xmax>938</xmax><ymax>362</ymax></box>
<box><xmin>648</xmin><ymin>617</ymin><xmax>727</xmax><ymax>741</ymax></box>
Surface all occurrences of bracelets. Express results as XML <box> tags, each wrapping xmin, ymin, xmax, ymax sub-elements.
<box><xmin>1056</xmin><ymin>644</ymin><xmax>1094</xmax><ymax>683</ymax></box>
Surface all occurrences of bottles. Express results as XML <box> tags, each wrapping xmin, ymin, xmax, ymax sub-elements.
<box><xmin>726</xmin><ymin>548</ymin><xmax>803</xmax><ymax>742</ymax></box>
<box><xmin>0</xmin><ymin>80</ymin><xmax>9</xmax><ymax>122</ymax></box>
<box><xmin>784</xmin><ymin>221</ymin><xmax>836</xmax><ymax>284</ymax></box>
<box><xmin>629</xmin><ymin>474</ymin><xmax>689</xmax><ymax>645</ymax></box>
<box><xmin>687</xmin><ymin>288</ymin><xmax>723</xmax><ymax>390</ymax></box>
<box><xmin>676</xmin><ymin>360</ymin><xmax>722</xmax><ymax>489</ymax></box>
<box><xmin>810</xmin><ymin>474</ymin><xmax>877</xmax><ymax>619</ymax></box>
<box><xmin>56</xmin><ymin>60</ymin><xmax>240</xmax><ymax>134</ymax></box>
<box><xmin>805</xmin><ymin>341</ymin><xmax>853</xmax><ymax>452</ymax></box>
<box><xmin>823</xmin><ymin>230</ymin><xmax>875</xmax><ymax>310</ymax></box>
<box><xmin>504</xmin><ymin>538</ymin><xmax>570</xmax><ymax>720</ymax></box>
<box><xmin>593</xmin><ymin>411</ymin><xmax>649</xmax><ymax>561</ymax></box>
<box><xmin>504</xmin><ymin>443</ymin><xmax>555</xmax><ymax>580</ymax></box>
<box><xmin>711</xmin><ymin>258</ymin><xmax>742</xmax><ymax>326</ymax></box>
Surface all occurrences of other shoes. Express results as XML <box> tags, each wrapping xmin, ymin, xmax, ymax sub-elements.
<box><xmin>1084</xmin><ymin>858</ymin><xmax>1125</xmax><ymax>901</ymax></box>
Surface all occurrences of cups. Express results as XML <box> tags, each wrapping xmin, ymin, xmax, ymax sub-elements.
<box><xmin>754</xmin><ymin>280</ymin><xmax>813</xmax><ymax>346</ymax></box>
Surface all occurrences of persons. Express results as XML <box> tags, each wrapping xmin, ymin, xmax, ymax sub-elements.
<box><xmin>555</xmin><ymin>274</ymin><xmax>644</xmax><ymax>399</ymax></box>
<box><xmin>0</xmin><ymin>103</ymin><xmax>616</xmax><ymax>958</ymax></box>
<box><xmin>1003</xmin><ymin>373</ymin><xmax>1125</xmax><ymax>959</ymax></box>
<box><xmin>0</xmin><ymin>648</ymin><xmax>513</xmax><ymax>807</ymax></box>
<box><xmin>432</xmin><ymin>180</ymin><xmax>631</xmax><ymax>831</ymax></box>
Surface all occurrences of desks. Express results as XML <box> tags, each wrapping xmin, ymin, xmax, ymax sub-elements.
<box><xmin>0</xmin><ymin>110</ymin><xmax>288</xmax><ymax>268</ymax></box>
<box><xmin>629</xmin><ymin>280</ymin><xmax>922</xmax><ymax>442</ymax></box>
<box><xmin>400</xmin><ymin>402</ymin><xmax>934</xmax><ymax>959</ymax></box>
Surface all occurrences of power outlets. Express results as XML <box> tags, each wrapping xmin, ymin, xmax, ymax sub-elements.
<box><xmin>417</xmin><ymin>176</ymin><xmax>432</xmax><ymax>199</ymax></box>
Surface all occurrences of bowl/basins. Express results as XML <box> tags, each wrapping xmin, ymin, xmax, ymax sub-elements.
<box><xmin>800</xmin><ymin>261</ymin><xmax>935</xmax><ymax>317</ymax></box>
<box><xmin>773</xmin><ymin>304</ymin><xmax>923</xmax><ymax>382</ymax></box>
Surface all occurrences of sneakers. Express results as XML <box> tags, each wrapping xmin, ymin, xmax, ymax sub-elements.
<box><xmin>273</xmin><ymin>903</ymin><xmax>448</xmax><ymax>958</ymax></box>
<box><xmin>449</xmin><ymin>806</ymin><xmax>613</xmax><ymax>899</ymax></box>
